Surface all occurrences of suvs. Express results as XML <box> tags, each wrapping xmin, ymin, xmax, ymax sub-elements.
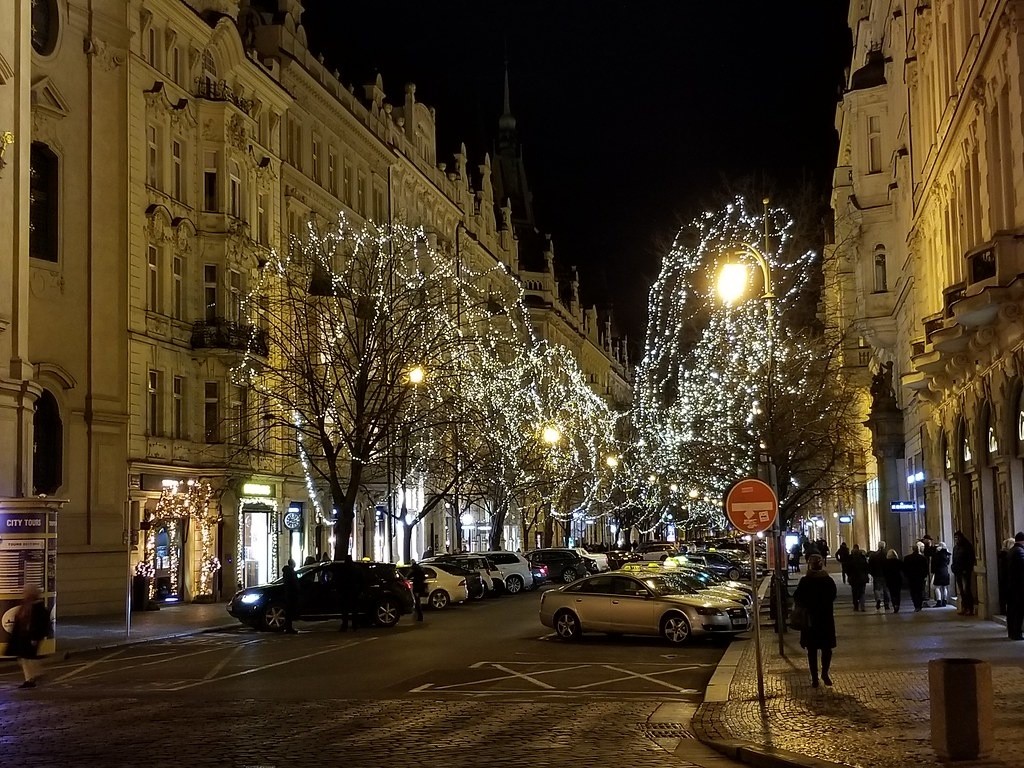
<box><xmin>226</xmin><ymin>560</ymin><xmax>415</xmax><ymax>630</ymax></box>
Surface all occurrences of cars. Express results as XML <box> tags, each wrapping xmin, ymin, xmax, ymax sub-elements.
<box><xmin>399</xmin><ymin>532</ymin><xmax>772</xmax><ymax>643</ymax></box>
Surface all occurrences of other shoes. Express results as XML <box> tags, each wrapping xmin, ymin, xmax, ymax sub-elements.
<box><xmin>18</xmin><ymin>680</ymin><xmax>38</xmax><ymax>690</ymax></box>
<box><xmin>934</xmin><ymin>599</ymin><xmax>948</xmax><ymax>607</ymax></box>
<box><xmin>812</xmin><ymin>675</ymin><xmax>833</xmax><ymax>686</ymax></box>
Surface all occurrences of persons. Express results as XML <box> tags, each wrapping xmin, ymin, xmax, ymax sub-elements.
<box><xmin>880</xmin><ymin>549</ymin><xmax>903</xmax><ymax>614</ymax></box>
<box><xmin>789</xmin><ymin>533</ymin><xmax>865</xmax><ymax>584</ymax></box>
<box><xmin>422</xmin><ymin>545</ymin><xmax>433</xmax><ymax>560</ymax></box>
<box><xmin>950</xmin><ymin>531</ymin><xmax>976</xmax><ymax>617</ymax></box>
<box><xmin>573</xmin><ymin>539</ymin><xmax>639</xmax><ymax>553</ymax></box>
<box><xmin>405</xmin><ymin>559</ymin><xmax>428</xmax><ymax>621</ymax></box>
<box><xmin>999</xmin><ymin>531</ymin><xmax>1024</xmax><ymax>641</ymax></box>
<box><xmin>332</xmin><ymin>555</ymin><xmax>362</xmax><ymax>632</ymax></box>
<box><xmin>516</xmin><ymin>548</ymin><xmax>521</xmax><ymax>553</ymax></box>
<box><xmin>845</xmin><ymin>544</ymin><xmax>870</xmax><ymax>611</ymax></box>
<box><xmin>792</xmin><ymin>554</ymin><xmax>837</xmax><ymax>688</ymax></box>
<box><xmin>1</xmin><ymin>585</ymin><xmax>55</xmax><ymax>691</ymax></box>
<box><xmin>281</xmin><ymin>559</ymin><xmax>301</xmax><ymax>634</ymax></box>
<box><xmin>917</xmin><ymin>534</ymin><xmax>937</xmax><ymax>608</ymax></box>
<box><xmin>930</xmin><ymin>541</ymin><xmax>951</xmax><ymax>608</ymax></box>
<box><xmin>867</xmin><ymin>541</ymin><xmax>892</xmax><ymax>611</ymax></box>
<box><xmin>304</xmin><ymin>556</ymin><xmax>317</xmax><ymax>582</ymax></box>
<box><xmin>320</xmin><ymin>551</ymin><xmax>332</xmax><ymax>582</ymax></box>
<box><xmin>902</xmin><ymin>545</ymin><xmax>929</xmax><ymax>613</ymax></box>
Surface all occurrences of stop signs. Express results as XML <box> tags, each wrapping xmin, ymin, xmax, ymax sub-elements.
<box><xmin>722</xmin><ymin>479</ymin><xmax>777</xmax><ymax>536</ymax></box>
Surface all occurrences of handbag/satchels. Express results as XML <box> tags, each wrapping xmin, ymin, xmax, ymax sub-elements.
<box><xmin>788</xmin><ymin>607</ymin><xmax>808</xmax><ymax>629</ymax></box>
<box><xmin>35</xmin><ymin>636</ymin><xmax>56</xmax><ymax>656</ymax></box>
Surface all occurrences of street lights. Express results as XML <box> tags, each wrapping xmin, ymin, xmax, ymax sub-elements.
<box><xmin>711</xmin><ymin>195</ymin><xmax>791</xmax><ymax>656</ymax></box>
